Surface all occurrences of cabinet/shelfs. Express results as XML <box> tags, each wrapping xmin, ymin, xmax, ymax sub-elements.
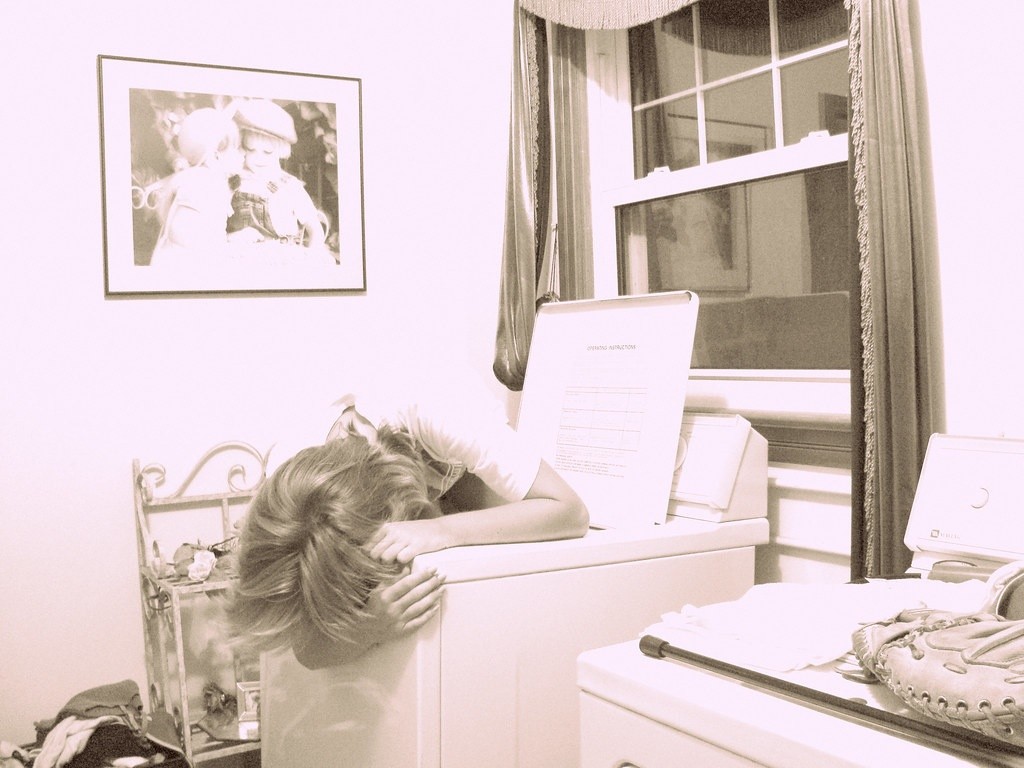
<box><xmin>133</xmin><ymin>441</ymin><xmax>277</xmax><ymax>768</ymax></box>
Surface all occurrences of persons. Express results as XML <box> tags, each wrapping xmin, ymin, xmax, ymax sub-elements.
<box><xmin>146</xmin><ymin>107</ymin><xmax>263</xmax><ymax>270</ymax></box>
<box><xmin>220</xmin><ymin>398</ymin><xmax>592</xmax><ymax>670</ymax></box>
<box><xmin>231</xmin><ymin>96</ymin><xmax>327</xmax><ymax>252</ymax></box>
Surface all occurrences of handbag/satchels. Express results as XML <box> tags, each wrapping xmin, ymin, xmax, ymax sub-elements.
<box><xmin>852</xmin><ymin>564</ymin><xmax>1024</xmax><ymax>748</ymax></box>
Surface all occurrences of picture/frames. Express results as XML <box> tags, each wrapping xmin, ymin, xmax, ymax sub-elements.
<box><xmin>653</xmin><ymin>113</ymin><xmax>768</xmax><ymax>292</ymax></box>
<box><xmin>97</xmin><ymin>54</ymin><xmax>367</xmax><ymax>296</ymax></box>
